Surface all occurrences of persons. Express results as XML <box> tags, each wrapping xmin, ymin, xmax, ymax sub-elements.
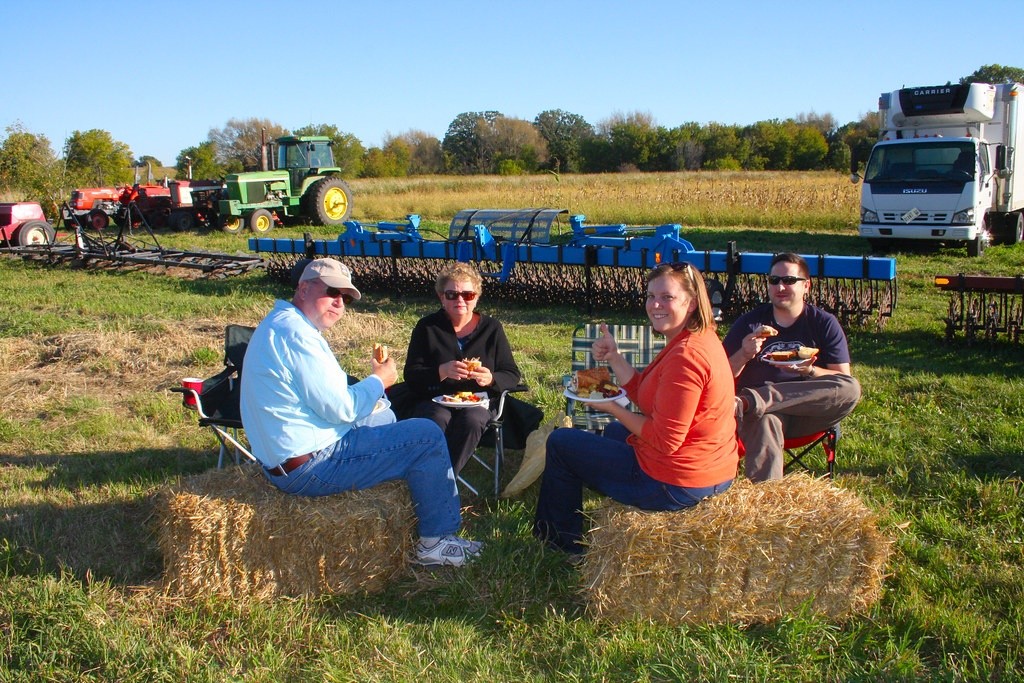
<box><xmin>534</xmin><ymin>260</ymin><xmax>739</xmax><ymax>566</ymax></box>
<box><xmin>403</xmin><ymin>262</ymin><xmax>521</xmax><ymax>479</ymax></box>
<box><xmin>723</xmin><ymin>252</ymin><xmax>861</xmax><ymax>484</ymax></box>
<box><xmin>51</xmin><ymin>200</ymin><xmax>59</xmax><ymax>214</ymax></box>
<box><xmin>239</xmin><ymin>258</ymin><xmax>486</xmax><ymax>569</ymax></box>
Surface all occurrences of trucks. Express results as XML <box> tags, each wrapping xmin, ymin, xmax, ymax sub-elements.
<box><xmin>850</xmin><ymin>82</ymin><xmax>1024</xmax><ymax>257</ymax></box>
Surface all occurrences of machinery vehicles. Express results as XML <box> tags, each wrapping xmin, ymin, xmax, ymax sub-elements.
<box><xmin>63</xmin><ymin>156</ymin><xmax>225</xmax><ymax>232</ymax></box>
<box><xmin>0</xmin><ymin>201</ymin><xmax>58</xmax><ymax>248</ymax></box>
<box><xmin>219</xmin><ymin>128</ymin><xmax>353</xmax><ymax>235</ymax></box>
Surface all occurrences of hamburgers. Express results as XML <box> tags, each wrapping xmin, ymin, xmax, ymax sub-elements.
<box><xmin>372</xmin><ymin>343</ymin><xmax>388</xmax><ymax>364</ymax></box>
<box><xmin>572</xmin><ymin>365</ymin><xmax>611</xmax><ymax>391</ymax></box>
<box><xmin>462</xmin><ymin>356</ymin><xmax>481</xmax><ymax>380</ymax></box>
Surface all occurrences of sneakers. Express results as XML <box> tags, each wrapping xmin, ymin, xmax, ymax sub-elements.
<box><xmin>417</xmin><ymin>536</ymin><xmax>487</xmax><ymax>566</ymax></box>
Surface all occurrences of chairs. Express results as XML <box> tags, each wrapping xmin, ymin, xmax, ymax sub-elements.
<box><xmin>783</xmin><ymin>422</ymin><xmax>839</xmax><ymax>486</ymax></box>
<box><xmin>946</xmin><ymin>160</ymin><xmax>974</xmax><ymax>178</ymax></box>
<box><xmin>458</xmin><ymin>384</ymin><xmax>528</xmax><ymax>497</ymax></box>
<box><xmin>169</xmin><ymin>325</ymin><xmax>258</xmax><ymax>470</ymax></box>
<box><xmin>891</xmin><ymin>162</ymin><xmax>915</xmax><ymax>178</ymax></box>
<box><xmin>302</xmin><ymin>152</ymin><xmax>321</xmax><ymax>167</ymax></box>
<box><xmin>566</xmin><ymin>322</ymin><xmax>666</xmax><ymax>437</ymax></box>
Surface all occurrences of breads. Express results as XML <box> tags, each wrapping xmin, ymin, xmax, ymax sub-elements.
<box><xmin>798</xmin><ymin>346</ymin><xmax>820</xmax><ymax>359</ymax></box>
<box><xmin>770</xmin><ymin>351</ymin><xmax>795</xmax><ymax>361</ymax></box>
<box><xmin>754</xmin><ymin>325</ymin><xmax>778</xmax><ymax>336</ymax></box>
<box><xmin>442</xmin><ymin>391</ymin><xmax>473</xmax><ymax>404</ymax></box>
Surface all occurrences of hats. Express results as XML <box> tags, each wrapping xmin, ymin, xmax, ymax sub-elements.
<box><xmin>299</xmin><ymin>258</ymin><xmax>361</xmax><ymax>300</ymax></box>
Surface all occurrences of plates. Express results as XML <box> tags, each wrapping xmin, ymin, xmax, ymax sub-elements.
<box><xmin>563</xmin><ymin>386</ymin><xmax>626</xmax><ymax>404</ymax></box>
<box><xmin>432</xmin><ymin>395</ymin><xmax>491</xmax><ymax>407</ymax></box>
<box><xmin>762</xmin><ymin>355</ymin><xmax>811</xmax><ymax>365</ymax></box>
<box><xmin>371</xmin><ymin>397</ymin><xmax>391</xmax><ymax>414</ymax></box>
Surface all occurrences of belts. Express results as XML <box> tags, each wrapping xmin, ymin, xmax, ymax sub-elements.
<box><xmin>264</xmin><ymin>452</ymin><xmax>313</xmax><ymax>476</ymax></box>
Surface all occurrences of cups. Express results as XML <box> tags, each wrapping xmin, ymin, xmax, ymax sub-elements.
<box><xmin>183</xmin><ymin>377</ymin><xmax>204</xmax><ymax>406</ymax></box>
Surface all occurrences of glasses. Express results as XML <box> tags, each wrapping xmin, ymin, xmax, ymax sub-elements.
<box><xmin>655</xmin><ymin>262</ymin><xmax>693</xmax><ymax>279</ymax></box>
<box><xmin>444</xmin><ymin>290</ymin><xmax>476</xmax><ymax>300</ymax></box>
<box><xmin>312</xmin><ymin>281</ymin><xmax>354</xmax><ymax>303</ymax></box>
<box><xmin>768</xmin><ymin>276</ymin><xmax>806</xmax><ymax>285</ymax></box>
